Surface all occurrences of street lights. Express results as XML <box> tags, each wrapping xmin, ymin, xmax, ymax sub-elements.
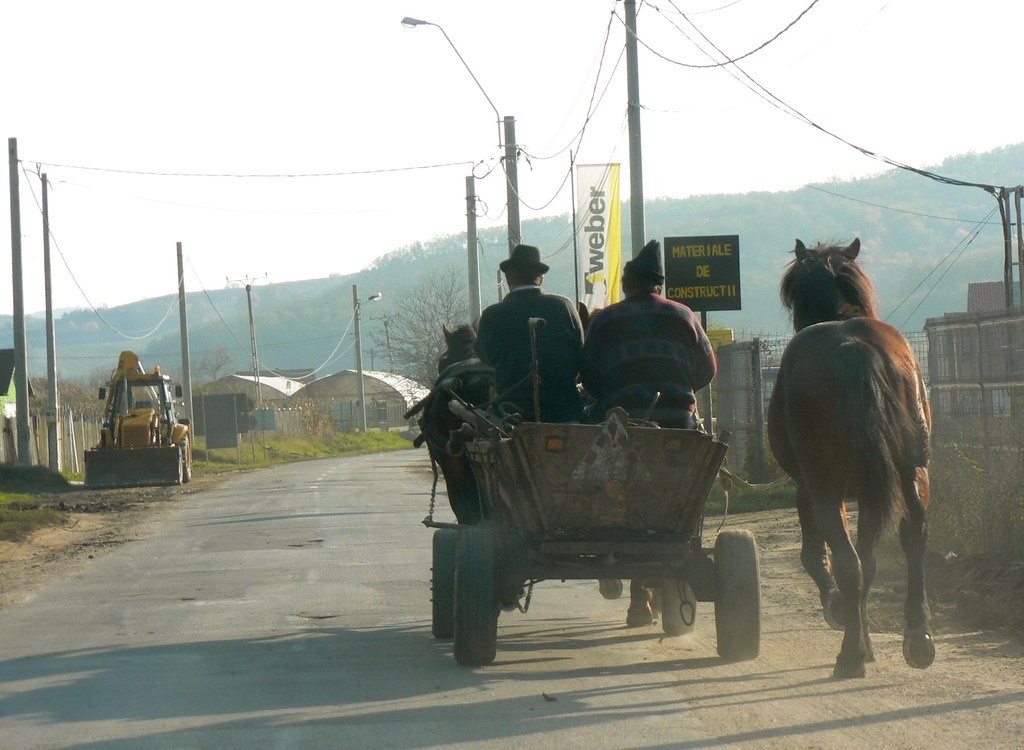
<box><xmin>402</xmin><ymin>15</ymin><xmax>523</xmax><ymax>256</ymax></box>
<box><xmin>353</xmin><ymin>283</ymin><xmax>383</xmax><ymax>433</ymax></box>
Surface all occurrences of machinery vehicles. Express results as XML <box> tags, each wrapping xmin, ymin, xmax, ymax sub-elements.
<box><xmin>84</xmin><ymin>349</ymin><xmax>192</xmax><ymax>492</ymax></box>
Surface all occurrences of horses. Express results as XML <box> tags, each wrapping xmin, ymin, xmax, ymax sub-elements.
<box><xmin>769</xmin><ymin>236</ymin><xmax>937</xmax><ymax>681</ymax></box>
<box><xmin>422</xmin><ymin>302</ymin><xmax>662</xmax><ymax>627</ymax></box>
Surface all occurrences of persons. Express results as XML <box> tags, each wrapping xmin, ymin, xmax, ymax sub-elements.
<box><xmin>473</xmin><ymin>244</ymin><xmax>584</xmax><ymax>424</ymax></box>
<box><xmin>584</xmin><ymin>240</ymin><xmax>717</xmax><ymax>430</ymax></box>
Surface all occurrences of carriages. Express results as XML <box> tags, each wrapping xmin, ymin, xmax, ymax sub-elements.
<box><xmin>402</xmin><ymin>316</ymin><xmax>762</xmax><ymax>668</ymax></box>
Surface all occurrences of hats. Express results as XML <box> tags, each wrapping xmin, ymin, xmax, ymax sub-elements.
<box><xmin>624</xmin><ymin>239</ymin><xmax>664</xmax><ymax>286</ymax></box>
<box><xmin>499</xmin><ymin>245</ymin><xmax>549</xmax><ymax>274</ymax></box>
